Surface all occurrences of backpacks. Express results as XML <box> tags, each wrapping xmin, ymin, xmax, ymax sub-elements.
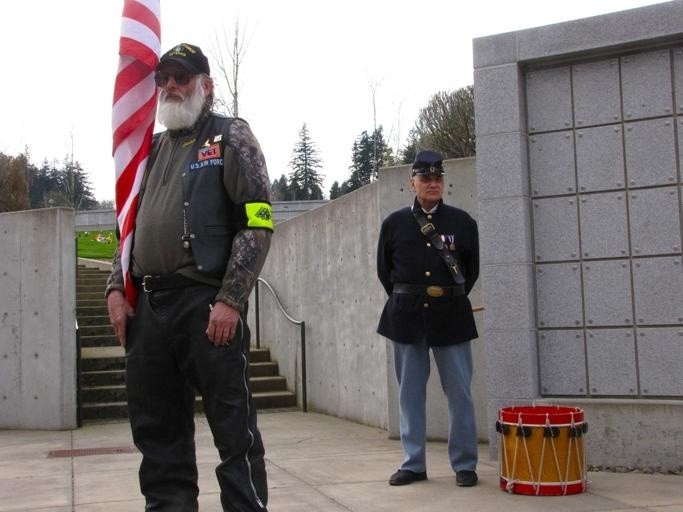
<box><xmin>495</xmin><ymin>407</ymin><xmax>587</xmax><ymax>496</ymax></box>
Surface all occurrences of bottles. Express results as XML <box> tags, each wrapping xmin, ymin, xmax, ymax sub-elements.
<box><xmin>156</xmin><ymin>43</ymin><xmax>210</xmax><ymax>76</ymax></box>
<box><xmin>412</xmin><ymin>151</ymin><xmax>445</xmax><ymax>176</ymax></box>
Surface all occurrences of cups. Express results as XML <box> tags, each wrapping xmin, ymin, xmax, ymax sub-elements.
<box><xmin>154</xmin><ymin>72</ymin><xmax>190</xmax><ymax>87</ymax></box>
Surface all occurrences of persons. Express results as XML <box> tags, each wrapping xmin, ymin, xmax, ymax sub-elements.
<box><xmin>103</xmin><ymin>40</ymin><xmax>276</xmax><ymax>512</ymax></box>
<box><xmin>375</xmin><ymin>150</ymin><xmax>482</xmax><ymax>486</ymax></box>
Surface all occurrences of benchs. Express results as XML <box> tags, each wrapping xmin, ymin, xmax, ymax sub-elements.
<box><xmin>112</xmin><ymin>0</ymin><xmax>160</xmax><ymax>312</ymax></box>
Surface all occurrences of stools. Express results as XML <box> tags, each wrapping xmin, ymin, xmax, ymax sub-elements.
<box><xmin>131</xmin><ymin>275</ymin><xmax>197</xmax><ymax>293</ymax></box>
<box><xmin>393</xmin><ymin>284</ymin><xmax>465</xmax><ymax>297</ymax></box>
<box><xmin>411</xmin><ymin>204</ymin><xmax>466</xmax><ymax>285</ymax></box>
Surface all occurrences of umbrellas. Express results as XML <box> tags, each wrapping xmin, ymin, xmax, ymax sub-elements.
<box><xmin>456</xmin><ymin>470</ymin><xmax>478</xmax><ymax>487</ymax></box>
<box><xmin>389</xmin><ymin>469</ymin><xmax>427</xmax><ymax>485</ymax></box>
